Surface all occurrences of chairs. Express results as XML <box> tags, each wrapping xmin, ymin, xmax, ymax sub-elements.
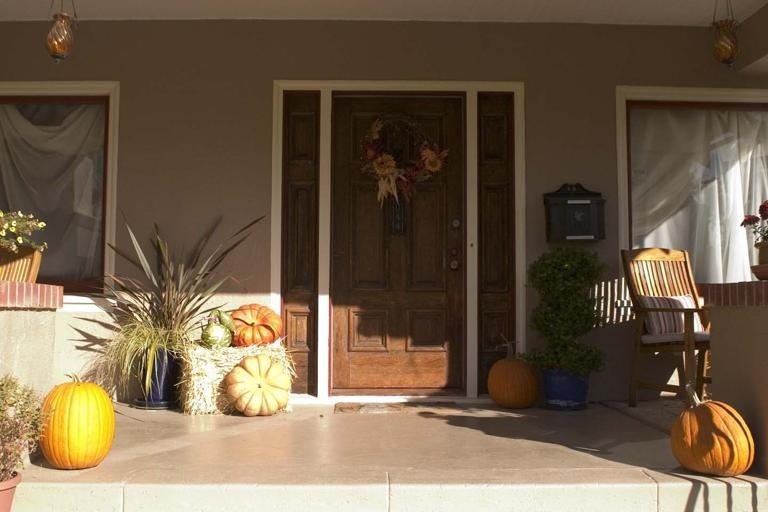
<box><xmin>617</xmin><ymin>244</ymin><xmax>714</xmax><ymax>407</ymax></box>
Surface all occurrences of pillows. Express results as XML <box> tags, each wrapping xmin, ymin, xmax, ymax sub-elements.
<box><xmin>632</xmin><ymin>288</ymin><xmax>707</xmax><ymax>338</ymax></box>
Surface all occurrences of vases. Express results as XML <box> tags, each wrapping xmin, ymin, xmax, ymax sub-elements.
<box><xmin>751</xmin><ymin>241</ymin><xmax>768</xmax><ymax>266</ymax></box>
<box><xmin>0</xmin><ymin>244</ymin><xmax>45</xmax><ymax>285</ymax></box>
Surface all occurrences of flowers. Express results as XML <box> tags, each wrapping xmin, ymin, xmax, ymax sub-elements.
<box><xmin>741</xmin><ymin>200</ymin><xmax>768</xmax><ymax>245</ymax></box>
<box><xmin>0</xmin><ymin>208</ymin><xmax>49</xmax><ymax>258</ymax></box>
<box><xmin>357</xmin><ymin>109</ymin><xmax>451</xmax><ymax>212</ymax></box>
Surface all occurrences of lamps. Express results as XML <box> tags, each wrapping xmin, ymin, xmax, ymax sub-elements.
<box><xmin>703</xmin><ymin>0</ymin><xmax>742</xmax><ymax>73</ymax></box>
<box><xmin>41</xmin><ymin>0</ymin><xmax>85</xmax><ymax>68</ymax></box>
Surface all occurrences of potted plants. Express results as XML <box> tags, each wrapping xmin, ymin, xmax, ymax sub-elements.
<box><xmin>76</xmin><ymin>207</ymin><xmax>267</xmax><ymax>412</ymax></box>
<box><xmin>512</xmin><ymin>244</ymin><xmax>613</xmax><ymax>413</ymax></box>
<box><xmin>0</xmin><ymin>371</ymin><xmax>57</xmax><ymax>511</ymax></box>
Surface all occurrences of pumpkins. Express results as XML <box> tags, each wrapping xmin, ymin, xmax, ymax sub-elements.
<box><xmin>229</xmin><ymin>303</ymin><xmax>282</xmax><ymax>348</ymax></box>
<box><xmin>670</xmin><ymin>384</ymin><xmax>755</xmax><ymax>477</ymax></box>
<box><xmin>485</xmin><ymin>334</ymin><xmax>539</xmax><ymax>409</ymax></box>
<box><xmin>226</xmin><ymin>354</ymin><xmax>290</xmax><ymax>417</ymax></box>
<box><xmin>35</xmin><ymin>373</ymin><xmax>115</xmax><ymax>469</ymax></box>
